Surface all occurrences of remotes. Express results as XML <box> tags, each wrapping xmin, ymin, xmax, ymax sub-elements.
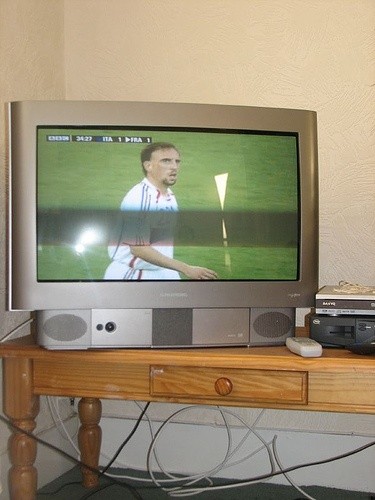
<box><xmin>285</xmin><ymin>337</ymin><xmax>322</xmax><ymax>357</ymax></box>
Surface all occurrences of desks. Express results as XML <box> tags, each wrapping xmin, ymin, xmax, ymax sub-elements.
<box><xmin>0</xmin><ymin>335</ymin><xmax>375</xmax><ymax>500</ymax></box>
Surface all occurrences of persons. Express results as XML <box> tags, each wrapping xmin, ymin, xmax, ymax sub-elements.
<box><xmin>103</xmin><ymin>141</ymin><xmax>218</xmax><ymax>281</ymax></box>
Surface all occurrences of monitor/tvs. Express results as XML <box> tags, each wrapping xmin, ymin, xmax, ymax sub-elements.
<box><xmin>6</xmin><ymin>100</ymin><xmax>320</xmax><ymax>350</ymax></box>
